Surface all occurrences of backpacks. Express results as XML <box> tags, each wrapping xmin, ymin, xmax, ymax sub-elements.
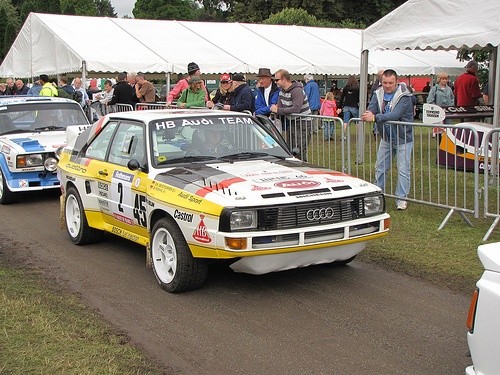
<box><xmin>73</xmin><ymin>90</ymin><xmax>84</xmax><ymax>102</ymax></box>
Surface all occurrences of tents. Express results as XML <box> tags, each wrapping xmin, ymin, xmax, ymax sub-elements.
<box><xmin>0</xmin><ymin>11</ymin><xmax>488</xmax><ymax>105</ymax></box>
<box><xmin>355</xmin><ymin>0</ymin><xmax>500</xmax><ymax>185</ymax></box>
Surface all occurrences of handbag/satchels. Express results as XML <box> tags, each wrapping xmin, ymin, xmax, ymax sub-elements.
<box><xmin>155</xmin><ymin>95</ymin><xmax>160</xmax><ymax>102</ymax></box>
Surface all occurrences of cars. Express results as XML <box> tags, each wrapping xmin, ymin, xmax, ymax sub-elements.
<box><xmin>55</xmin><ymin>108</ymin><xmax>392</xmax><ymax>295</ymax></box>
<box><xmin>463</xmin><ymin>242</ymin><xmax>500</xmax><ymax>375</ymax></box>
<box><xmin>0</xmin><ymin>93</ymin><xmax>103</xmax><ymax>207</ymax></box>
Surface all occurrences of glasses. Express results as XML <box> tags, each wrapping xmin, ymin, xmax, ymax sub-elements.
<box><xmin>274</xmin><ymin>79</ymin><xmax>281</xmax><ymax>82</ymax></box>
<box><xmin>220</xmin><ymin>81</ymin><xmax>230</xmax><ymax>83</ymax></box>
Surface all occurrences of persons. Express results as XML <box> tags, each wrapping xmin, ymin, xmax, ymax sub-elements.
<box><xmin>425</xmin><ymin>72</ymin><xmax>455</xmax><ymax>148</ymax></box>
<box><xmin>319</xmin><ymin>92</ymin><xmax>340</xmax><ymax>141</ymax></box>
<box><xmin>482</xmin><ymin>82</ymin><xmax>488</xmax><ymax>106</ymax></box>
<box><xmin>361</xmin><ymin>68</ymin><xmax>415</xmax><ymax>212</ymax></box>
<box><xmin>189</xmin><ymin>124</ymin><xmax>242</xmax><ymax>159</ymax></box>
<box><xmin>336</xmin><ymin>74</ymin><xmax>361</xmax><ymax>141</ymax></box>
<box><xmin>0</xmin><ymin>61</ymin><xmax>432</xmax><ymax>115</ymax></box>
<box><xmin>453</xmin><ymin>59</ymin><xmax>482</xmax><ymax>124</ymax></box>
<box><xmin>268</xmin><ymin>69</ymin><xmax>313</xmax><ymax>163</ymax></box>
<box><xmin>302</xmin><ymin>74</ymin><xmax>321</xmax><ymax>136</ymax></box>
<box><xmin>250</xmin><ymin>68</ymin><xmax>283</xmax><ymax>137</ymax></box>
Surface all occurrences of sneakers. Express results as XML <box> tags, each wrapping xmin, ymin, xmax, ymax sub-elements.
<box><xmin>396</xmin><ymin>198</ymin><xmax>408</xmax><ymax>209</ymax></box>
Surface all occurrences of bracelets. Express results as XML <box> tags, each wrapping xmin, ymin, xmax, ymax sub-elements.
<box><xmin>372</xmin><ymin>115</ymin><xmax>375</xmax><ymax>122</ymax></box>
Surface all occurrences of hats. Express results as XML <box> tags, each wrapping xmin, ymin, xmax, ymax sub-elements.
<box><xmin>256</xmin><ymin>68</ymin><xmax>273</xmax><ymax>78</ymax></box>
<box><xmin>188</xmin><ymin>62</ymin><xmax>200</xmax><ymax>75</ymax></box>
<box><xmin>220</xmin><ymin>74</ymin><xmax>231</xmax><ymax>81</ymax></box>
<box><xmin>188</xmin><ymin>76</ymin><xmax>203</xmax><ymax>84</ymax></box>
<box><xmin>231</xmin><ymin>75</ymin><xmax>245</xmax><ymax>82</ymax></box>
